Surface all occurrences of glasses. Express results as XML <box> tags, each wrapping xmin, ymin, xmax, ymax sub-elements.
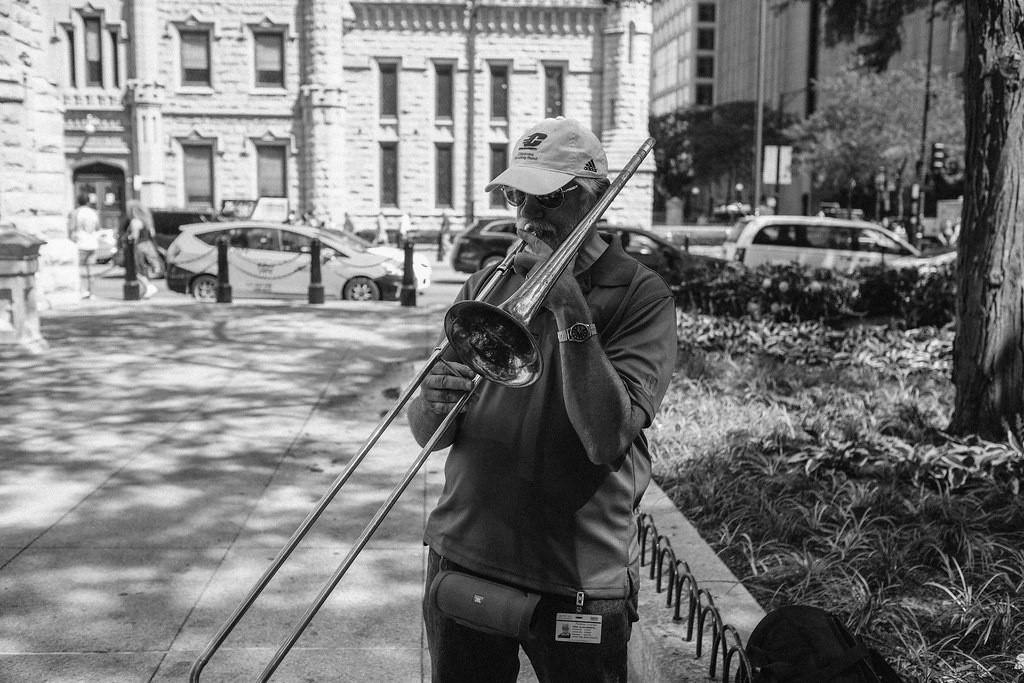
<box><xmin>501</xmin><ymin>185</ymin><xmax>581</xmax><ymax>210</ymax></box>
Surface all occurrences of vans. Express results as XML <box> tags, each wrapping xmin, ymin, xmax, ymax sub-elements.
<box><xmin>723</xmin><ymin>215</ymin><xmax>919</xmax><ymax>269</ymax></box>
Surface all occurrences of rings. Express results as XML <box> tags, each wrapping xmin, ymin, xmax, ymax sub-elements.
<box><xmin>526</xmin><ymin>273</ymin><xmax>529</xmax><ymax>281</ymax></box>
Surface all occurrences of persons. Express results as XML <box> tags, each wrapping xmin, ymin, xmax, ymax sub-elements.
<box><xmin>695</xmin><ymin>192</ymin><xmax>960</xmax><ymax>255</ymax></box>
<box><xmin>557</xmin><ymin>623</ymin><xmax>571</xmax><ymax>638</ymax></box>
<box><xmin>283</xmin><ymin>210</ymin><xmax>454</xmax><ymax>266</ymax></box>
<box><xmin>121</xmin><ymin>201</ymin><xmax>160</xmax><ymax>298</ymax></box>
<box><xmin>67</xmin><ymin>192</ymin><xmax>101</xmax><ymax>298</ymax></box>
<box><xmin>407</xmin><ymin>115</ymin><xmax>678</xmax><ymax>683</ymax></box>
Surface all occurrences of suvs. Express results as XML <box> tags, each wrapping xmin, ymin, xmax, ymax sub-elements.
<box><xmin>165</xmin><ymin>220</ymin><xmax>432</xmax><ymax>302</ymax></box>
<box><xmin>451</xmin><ymin>218</ymin><xmax>519</xmax><ymax>274</ymax></box>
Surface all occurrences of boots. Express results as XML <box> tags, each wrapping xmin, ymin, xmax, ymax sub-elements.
<box><xmin>140</xmin><ymin>276</ymin><xmax>157</xmax><ymax>299</ymax></box>
<box><xmin>136</xmin><ymin>273</ymin><xmax>145</xmax><ymax>297</ymax></box>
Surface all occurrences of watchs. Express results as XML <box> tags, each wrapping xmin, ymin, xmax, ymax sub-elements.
<box><xmin>557</xmin><ymin>321</ymin><xmax>598</xmax><ymax>343</ymax></box>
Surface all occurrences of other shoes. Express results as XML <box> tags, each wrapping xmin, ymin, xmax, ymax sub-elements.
<box><xmin>81</xmin><ymin>290</ymin><xmax>90</xmax><ymax>299</ymax></box>
<box><xmin>90</xmin><ymin>295</ymin><xmax>96</xmax><ymax>300</ymax></box>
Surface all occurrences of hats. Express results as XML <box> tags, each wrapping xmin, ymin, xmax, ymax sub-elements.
<box><xmin>485</xmin><ymin>115</ymin><xmax>607</xmax><ymax>196</ymax></box>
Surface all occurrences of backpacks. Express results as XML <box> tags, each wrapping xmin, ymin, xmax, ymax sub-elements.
<box><xmin>737</xmin><ymin>605</ymin><xmax>904</xmax><ymax>683</ymax></box>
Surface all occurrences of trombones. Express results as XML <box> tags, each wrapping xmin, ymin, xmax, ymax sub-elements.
<box><xmin>187</xmin><ymin>136</ymin><xmax>657</xmax><ymax>683</ymax></box>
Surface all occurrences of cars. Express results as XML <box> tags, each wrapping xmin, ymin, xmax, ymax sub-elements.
<box><xmin>596</xmin><ymin>223</ymin><xmax>729</xmax><ymax>286</ymax></box>
